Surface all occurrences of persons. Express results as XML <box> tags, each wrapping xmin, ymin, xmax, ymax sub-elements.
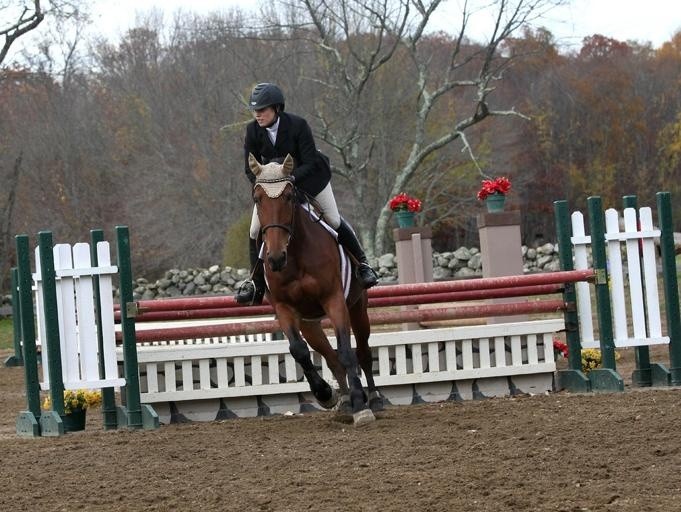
<box><xmin>235</xmin><ymin>83</ymin><xmax>379</xmax><ymax>303</ymax></box>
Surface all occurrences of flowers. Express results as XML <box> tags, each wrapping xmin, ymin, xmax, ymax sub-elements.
<box><xmin>41</xmin><ymin>388</ymin><xmax>103</xmax><ymax>414</ymax></box>
<box><xmin>388</xmin><ymin>191</ymin><xmax>422</xmax><ymax>213</ymax></box>
<box><xmin>477</xmin><ymin>176</ymin><xmax>512</xmax><ymax>201</ymax></box>
<box><xmin>553</xmin><ymin>339</ymin><xmax>622</xmax><ymax>378</ymax></box>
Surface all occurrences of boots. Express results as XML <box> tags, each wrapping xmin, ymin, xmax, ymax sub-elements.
<box><xmin>234</xmin><ymin>233</ymin><xmax>265</xmax><ymax>305</ymax></box>
<box><xmin>335</xmin><ymin>216</ymin><xmax>377</xmax><ymax>289</ymax></box>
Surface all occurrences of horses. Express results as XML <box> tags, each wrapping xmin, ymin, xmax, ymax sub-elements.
<box><xmin>245</xmin><ymin>151</ymin><xmax>384</xmax><ymax>430</ymax></box>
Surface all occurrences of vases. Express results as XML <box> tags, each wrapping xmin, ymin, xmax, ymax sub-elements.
<box><xmin>64</xmin><ymin>408</ymin><xmax>87</xmax><ymax>432</ymax></box>
<box><xmin>396</xmin><ymin>210</ymin><xmax>415</xmax><ymax>228</ymax></box>
<box><xmin>487</xmin><ymin>195</ymin><xmax>506</xmax><ymax>213</ymax></box>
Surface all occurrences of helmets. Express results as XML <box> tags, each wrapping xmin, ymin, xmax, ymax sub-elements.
<box><xmin>249</xmin><ymin>83</ymin><xmax>285</xmax><ymax>110</ymax></box>
